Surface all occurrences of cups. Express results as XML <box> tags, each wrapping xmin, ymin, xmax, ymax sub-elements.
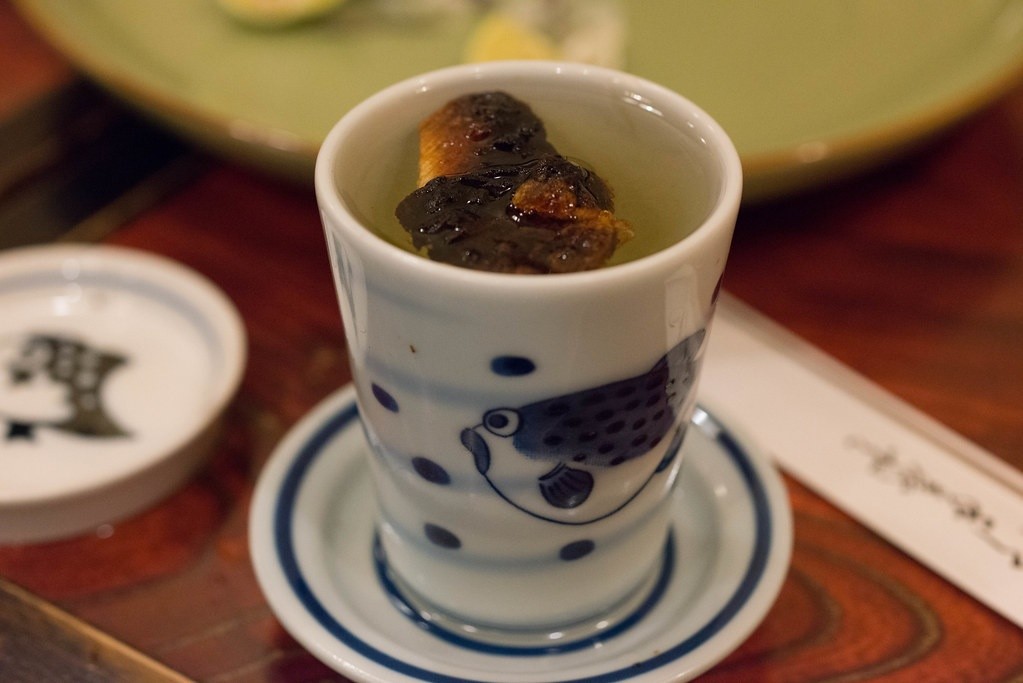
<box><xmin>313</xmin><ymin>61</ymin><xmax>743</xmax><ymax>634</ymax></box>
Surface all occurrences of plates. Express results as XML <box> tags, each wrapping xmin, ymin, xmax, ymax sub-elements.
<box><xmin>246</xmin><ymin>380</ymin><xmax>793</xmax><ymax>683</ymax></box>
<box><xmin>13</xmin><ymin>0</ymin><xmax>1023</xmax><ymax>203</ymax></box>
<box><xmin>0</xmin><ymin>241</ymin><xmax>248</xmax><ymax>546</ymax></box>
